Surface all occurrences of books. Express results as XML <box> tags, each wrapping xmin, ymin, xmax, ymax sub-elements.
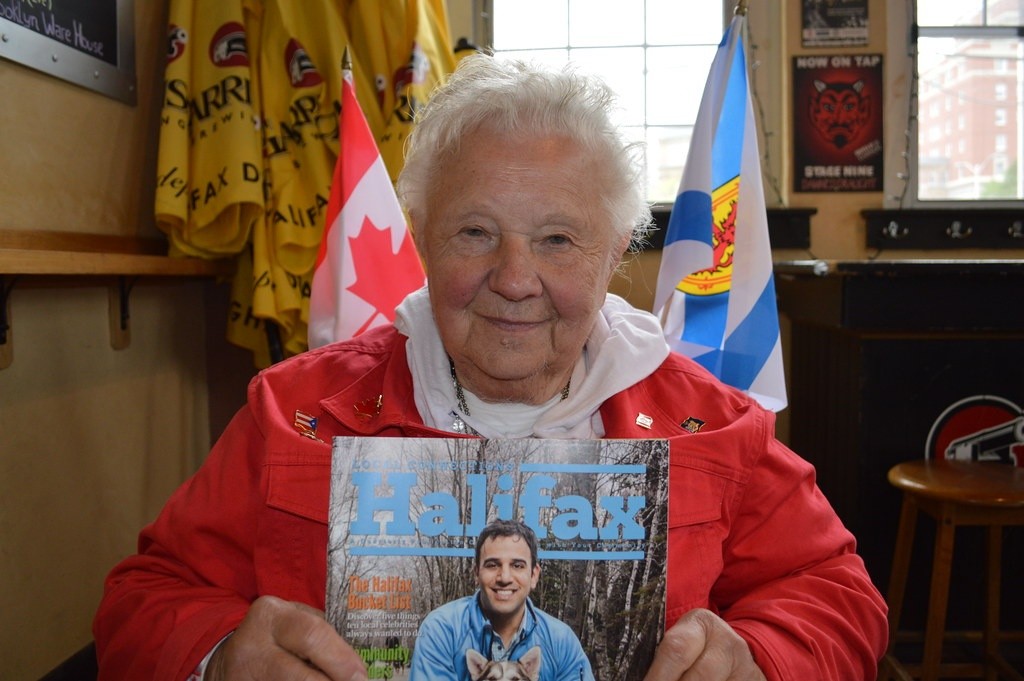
<box><xmin>324</xmin><ymin>437</ymin><xmax>670</xmax><ymax>680</ymax></box>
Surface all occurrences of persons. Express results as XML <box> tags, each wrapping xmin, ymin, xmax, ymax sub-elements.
<box><xmin>407</xmin><ymin>517</ymin><xmax>596</xmax><ymax>680</ymax></box>
<box><xmin>92</xmin><ymin>50</ymin><xmax>891</xmax><ymax>681</ymax></box>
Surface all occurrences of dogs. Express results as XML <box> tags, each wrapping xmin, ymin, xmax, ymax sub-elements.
<box><xmin>465</xmin><ymin>645</ymin><xmax>542</xmax><ymax>681</ymax></box>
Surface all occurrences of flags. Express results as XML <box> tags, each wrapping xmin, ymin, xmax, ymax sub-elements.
<box><xmin>306</xmin><ymin>75</ymin><xmax>427</xmax><ymax>351</ymax></box>
<box><xmin>648</xmin><ymin>15</ymin><xmax>788</xmax><ymax>412</ymax></box>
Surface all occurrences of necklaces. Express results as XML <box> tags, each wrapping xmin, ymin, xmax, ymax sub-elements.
<box><xmin>447</xmin><ymin>360</ymin><xmax>570</xmax><ymax>439</ymax></box>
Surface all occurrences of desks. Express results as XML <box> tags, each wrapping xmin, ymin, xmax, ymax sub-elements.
<box><xmin>774</xmin><ymin>260</ymin><xmax>1023</xmax><ymax>649</ymax></box>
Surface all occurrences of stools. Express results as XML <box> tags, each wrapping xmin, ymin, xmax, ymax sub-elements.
<box><xmin>880</xmin><ymin>460</ymin><xmax>1024</xmax><ymax>680</ymax></box>
